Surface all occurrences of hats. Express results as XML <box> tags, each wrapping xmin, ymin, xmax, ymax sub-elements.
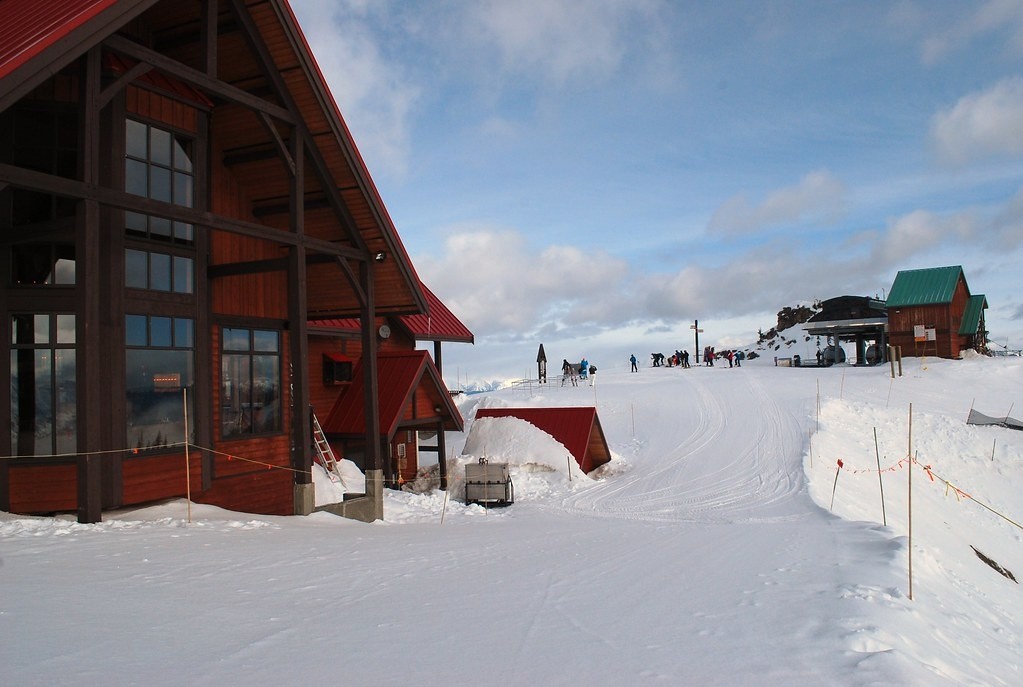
<box><xmin>563</xmin><ymin>359</ymin><xmax>566</xmax><ymax>361</ymax></box>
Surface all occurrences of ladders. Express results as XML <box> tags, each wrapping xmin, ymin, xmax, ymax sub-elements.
<box><xmin>312</xmin><ymin>412</ymin><xmax>348</xmax><ymax>491</ymax></box>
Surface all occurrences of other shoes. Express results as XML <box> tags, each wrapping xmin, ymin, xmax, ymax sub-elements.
<box><xmin>729</xmin><ymin>366</ymin><xmax>732</xmax><ymax>368</ymax></box>
<box><xmin>735</xmin><ymin>364</ymin><xmax>737</xmax><ymax>365</ymax></box>
<box><xmin>687</xmin><ymin>365</ymin><xmax>690</xmax><ymax>368</ymax></box>
<box><xmin>683</xmin><ymin>367</ymin><xmax>687</xmax><ymax>369</ymax></box>
<box><xmin>737</xmin><ymin>365</ymin><xmax>741</xmax><ymax>367</ymax></box>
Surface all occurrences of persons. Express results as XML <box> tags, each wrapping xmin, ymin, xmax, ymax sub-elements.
<box><xmin>704</xmin><ymin>346</ymin><xmax>741</xmax><ymax>368</ymax></box>
<box><xmin>651</xmin><ymin>349</ymin><xmax>690</xmax><ymax>368</ymax></box>
<box><xmin>560</xmin><ymin>356</ymin><xmax>597</xmax><ymax>386</ymax></box>
<box><xmin>816</xmin><ymin>350</ymin><xmax>823</xmax><ymax>365</ymax></box>
<box><xmin>630</xmin><ymin>354</ymin><xmax>637</xmax><ymax>372</ymax></box>
